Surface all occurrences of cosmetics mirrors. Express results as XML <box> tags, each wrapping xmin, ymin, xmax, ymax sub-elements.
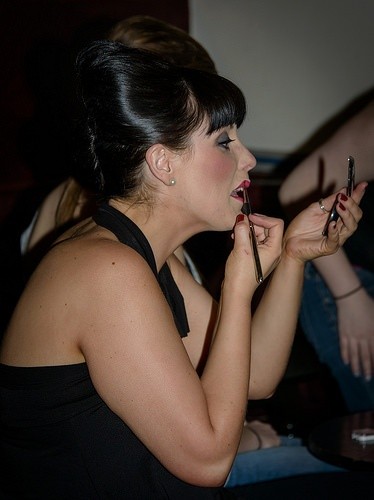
<box><xmin>321</xmin><ymin>155</ymin><xmax>355</xmax><ymax>236</ymax></box>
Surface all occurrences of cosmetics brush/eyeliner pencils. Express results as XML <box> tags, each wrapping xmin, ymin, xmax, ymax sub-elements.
<box><xmin>240</xmin><ymin>181</ymin><xmax>263</xmax><ymax>283</ymax></box>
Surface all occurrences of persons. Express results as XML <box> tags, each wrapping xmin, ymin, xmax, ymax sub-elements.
<box><xmin>20</xmin><ymin>18</ymin><xmax>223</xmax><ymax>287</ymax></box>
<box><xmin>270</xmin><ymin>88</ymin><xmax>373</xmax><ymax>414</ymax></box>
<box><xmin>1</xmin><ymin>39</ymin><xmax>368</xmax><ymax>499</ymax></box>
